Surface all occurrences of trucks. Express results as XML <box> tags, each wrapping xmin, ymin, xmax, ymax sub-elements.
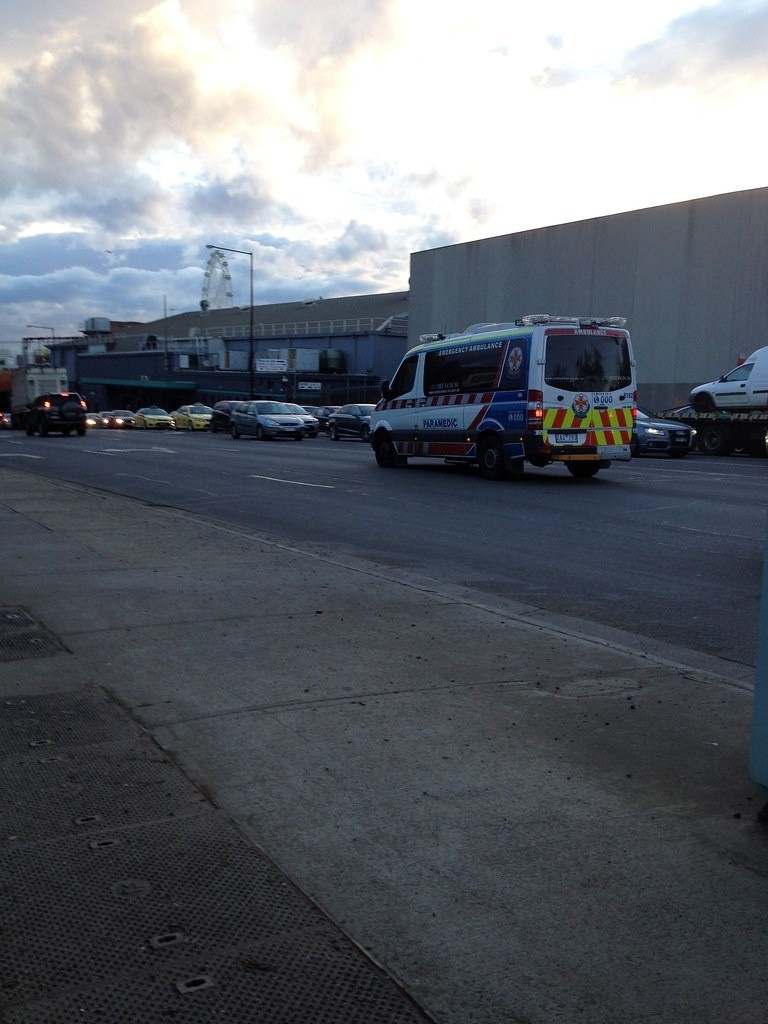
<box><xmin>9</xmin><ymin>366</ymin><xmax>69</xmax><ymax>429</ymax></box>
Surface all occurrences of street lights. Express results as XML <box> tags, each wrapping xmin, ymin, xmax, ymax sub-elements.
<box><xmin>206</xmin><ymin>244</ymin><xmax>255</xmax><ymax>401</ymax></box>
<box><xmin>27</xmin><ymin>324</ymin><xmax>57</xmax><ymax>372</ymax></box>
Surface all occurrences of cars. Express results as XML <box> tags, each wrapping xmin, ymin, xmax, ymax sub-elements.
<box><xmin>0</xmin><ymin>414</ymin><xmax>11</xmax><ymax>430</ymax></box>
<box><xmin>210</xmin><ymin>400</ymin><xmax>246</xmax><ymax>434</ymax></box>
<box><xmin>170</xmin><ymin>402</ymin><xmax>215</xmax><ymax>432</ymax></box>
<box><xmin>98</xmin><ymin>411</ymin><xmax>111</xmax><ymax>421</ymax></box>
<box><xmin>229</xmin><ymin>399</ymin><xmax>306</xmax><ymax>441</ymax></box>
<box><xmin>326</xmin><ymin>403</ymin><xmax>377</xmax><ymax>443</ymax></box>
<box><xmin>309</xmin><ymin>406</ymin><xmax>343</xmax><ymax>435</ymax></box>
<box><xmin>110</xmin><ymin>410</ymin><xmax>136</xmax><ymax>430</ymax></box>
<box><xmin>301</xmin><ymin>405</ymin><xmax>319</xmax><ymax>417</ymax></box>
<box><xmin>274</xmin><ymin>402</ymin><xmax>321</xmax><ymax>438</ymax></box>
<box><xmin>633</xmin><ymin>405</ymin><xmax>698</xmax><ymax>459</ymax></box>
<box><xmin>84</xmin><ymin>413</ymin><xmax>108</xmax><ymax>429</ymax></box>
<box><xmin>135</xmin><ymin>406</ymin><xmax>176</xmax><ymax>431</ymax></box>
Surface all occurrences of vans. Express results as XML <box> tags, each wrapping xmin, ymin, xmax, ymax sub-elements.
<box><xmin>688</xmin><ymin>345</ymin><xmax>768</xmax><ymax>413</ymax></box>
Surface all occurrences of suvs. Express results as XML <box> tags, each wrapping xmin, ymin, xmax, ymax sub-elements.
<box><xmin>25</xmin><ymin>392</ymin><xmax>88</xmax><ymax>436</ymax></box>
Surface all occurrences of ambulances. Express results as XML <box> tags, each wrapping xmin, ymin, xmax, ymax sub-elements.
<box><xmin>368</xmin><ymin>313</ymin><xmax>638</xmax><ymax>481</ymax></box>
